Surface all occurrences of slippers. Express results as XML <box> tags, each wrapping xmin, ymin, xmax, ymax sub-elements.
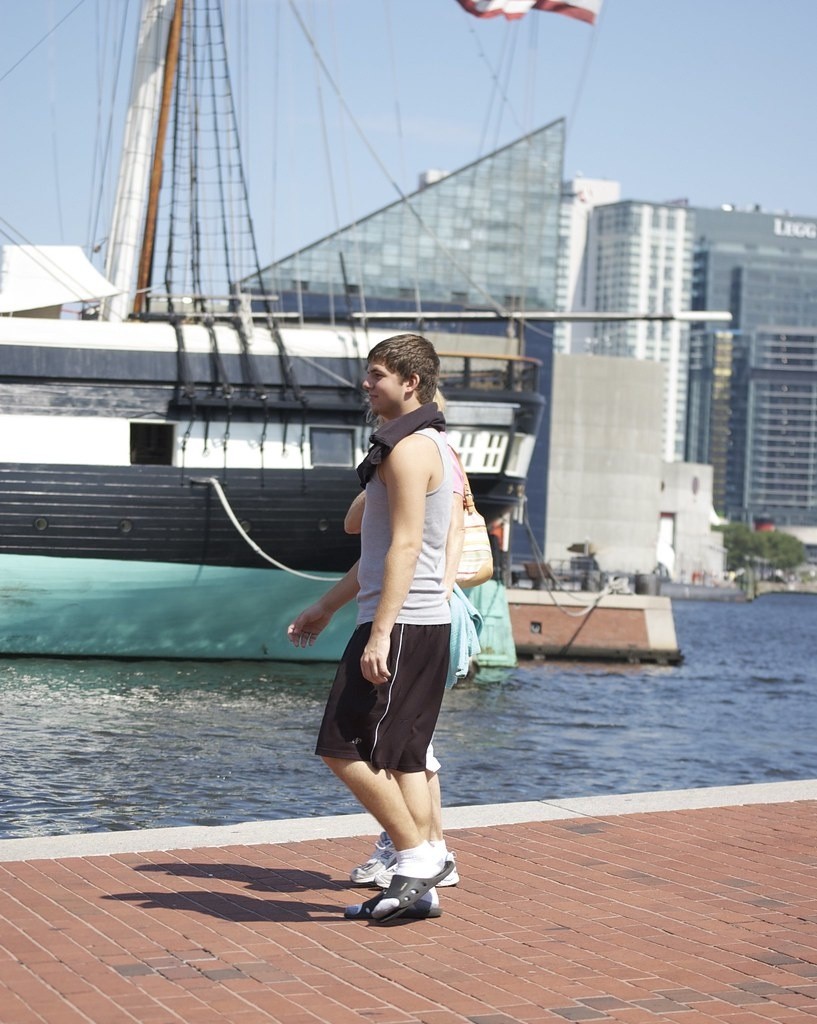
<box><xmin>344</xmin><ymin>888</ymin><xmax>441</xmax><ymax>918</ymax></box>
<box><xmin>376</xmin><ymin>861</ymin><xmax>455</xmax><ymax>924</ymax></box>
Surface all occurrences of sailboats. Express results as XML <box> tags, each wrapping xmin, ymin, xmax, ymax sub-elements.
<box><xmin>0</xmin><ymin>0</ymin><xmax>688</xmax><ymax>667</ymax></box>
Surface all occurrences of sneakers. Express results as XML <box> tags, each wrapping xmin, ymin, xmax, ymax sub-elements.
<box><xmin>374</xmin><ymin>849</ymin><xmax>460</xmax><ymax>886</ymax></box>
<box><xmin>351</xmin><ymin>833</ymin><xmax>398</xmax><ymax>884</ymax></box>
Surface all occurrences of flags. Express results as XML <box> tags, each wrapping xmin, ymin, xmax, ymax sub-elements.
<box><xmin>456</xmin><ymin>0</ymin><xmax>601</xmax><ymax>26</ymax></box>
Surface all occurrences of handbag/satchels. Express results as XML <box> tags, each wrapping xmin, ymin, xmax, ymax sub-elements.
<box><xmin>448</xmin><ymin>444</ymin><xmax>493</xmax><ymax>588</ymax></box>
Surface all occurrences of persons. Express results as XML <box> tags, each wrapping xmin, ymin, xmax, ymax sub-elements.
<box><xmin>285</xmin><ymin>333</ymin><xmax>493</xmax><ymax>926</ymax></box>
